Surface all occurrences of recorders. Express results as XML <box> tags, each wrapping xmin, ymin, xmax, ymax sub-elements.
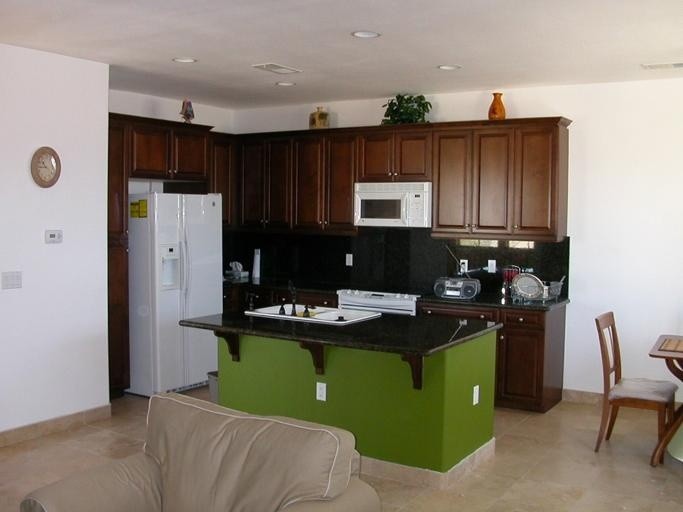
<box><xmin>433</xmin><ymin>277</ymin><xmax>481</xmax><ymax>300</ymax></box>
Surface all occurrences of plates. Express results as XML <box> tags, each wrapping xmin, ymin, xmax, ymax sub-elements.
<box><xmin>511</xmin><ymin>273</ymin><xmax>544</xmax><ymax>299</ymax></box>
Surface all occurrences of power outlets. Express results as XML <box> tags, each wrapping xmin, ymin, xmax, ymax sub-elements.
<box><xmin>459</xmin><ymin>258</ymin><xmax>466</xmax><ymax>274</ymax></box>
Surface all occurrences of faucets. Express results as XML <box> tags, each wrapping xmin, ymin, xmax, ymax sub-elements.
<box><xmin>248</xmin><ymin>292</ymin><xmax>259</xmax><ymax>312</ymax></box>
<box><xmin>288</xmin><ymin>280</ymin><xmax>298</xmax><ymax>316</ymax></box>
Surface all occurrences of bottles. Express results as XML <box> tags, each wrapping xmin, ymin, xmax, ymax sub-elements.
<box><xmin>252</xmin><ymin>279</ymin><xmax>259</xmax><ymax>285</ymax></box>
<box><xmin>505</xmin><ymin>299</ymin><xmax>513</xmax><ymax>305</ymax></box>
<box><xmin>504</xmin><ymin>268</ymin><xmax>519</xmax><ymax>299</ymax></box>
<box><xmin>251</xmin><ymin>247</ymin><xmax>261</xmax><ymax>280</ymax></box>
<box><xmin>542</xmin><ymin>280</ymin><xmax>550</xmax><ymax>297</ymax></box>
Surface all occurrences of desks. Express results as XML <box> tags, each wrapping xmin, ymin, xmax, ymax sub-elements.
<box><xmin>649</xmin><ymin>334</ymin><xmax>682</xmax><ymax>467</ymax></box>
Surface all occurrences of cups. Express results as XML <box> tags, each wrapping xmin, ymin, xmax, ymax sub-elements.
<box><xmin>549</xmin><ymin>281</ymin><xmax>562</xmax><ymax>296</ymax></box>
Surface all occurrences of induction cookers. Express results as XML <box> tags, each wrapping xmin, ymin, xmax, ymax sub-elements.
<box><xmin>333</xmin><ymin>283</ymin><xmax>433</xmax><ymax>308</ymax></box>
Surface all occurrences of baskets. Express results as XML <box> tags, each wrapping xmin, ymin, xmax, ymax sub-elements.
<box><xmin>511</xmin><ymin>282</ymin><xmax>562</xmax><ymax>300</ymax></box>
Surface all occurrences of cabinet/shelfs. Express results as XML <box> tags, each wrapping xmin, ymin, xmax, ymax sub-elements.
<box><xmin>107</xmin><ymin>112</ymin><xmax>128</xmax><ymax>245</ymax></box>
<box><xmin>222</xmin><ymin>274</ymin><xmax>337</xmax><ymax>318</ymax></box>
<box><xmin>356</xmin><ymin>123</ymin><xmax>430</xmax><ymax>182</ymax></box>
<box><xmin>416</xmin><ymin>297</ymin><xmax>569</xmax><ymax>413</ymax></box>
<box><xmin>108</xmin><ymin>246</ymin><xmax>129</xmax><ymax>401</ymax></box>
<box><xmin>431</xmin><ymin>116</ymin><xmax>571</xmax><ymax>243</ymax></box>
<box><xmin>129</xmin><ymin>113</ymin><xmax>214</xmax><ymax>180</ymax></box>
<box><xmin>210</xmin><ymin>131</ymin><xmax>355</xmax><ymax>234</ymax></box>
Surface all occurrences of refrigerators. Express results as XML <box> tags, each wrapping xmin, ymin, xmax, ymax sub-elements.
<box><xmin>127</xmin><ymin>192</ymin><xmax>223</xmax><ymax>399</ymax></box>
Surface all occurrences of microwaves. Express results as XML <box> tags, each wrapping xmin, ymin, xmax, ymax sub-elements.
<box><xmin>352</xmin><ymin>182</ymin><xmax>432</xmax><ymax>229</ymax></box>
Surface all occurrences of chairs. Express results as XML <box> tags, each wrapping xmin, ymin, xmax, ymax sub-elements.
<box><xmin>594</xmin><ymin>309</ymin><xmax>677</xmax><ymax>465</ymax></box>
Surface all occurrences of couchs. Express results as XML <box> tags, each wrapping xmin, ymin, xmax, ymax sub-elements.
<box><xmin>20</xmin><ymin>393</ymin><xmax>384</xmax><ymax>511</ymax></box>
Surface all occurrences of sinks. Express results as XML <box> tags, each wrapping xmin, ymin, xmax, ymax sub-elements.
<box><xmin>311</xmin><ymin>309</ymin><xmax>382</xmax><ymax>327</ymax></box>
<box><xmin>243</xmin><ymin>304</ymin><xmax>333</xmax><ymax>322</ymax></box>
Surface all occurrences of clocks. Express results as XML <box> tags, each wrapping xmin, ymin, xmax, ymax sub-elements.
<box><xmin>30</xmin><ymin>146</ymin><xmax>61</xmax><ymax>188</ymax></box>
